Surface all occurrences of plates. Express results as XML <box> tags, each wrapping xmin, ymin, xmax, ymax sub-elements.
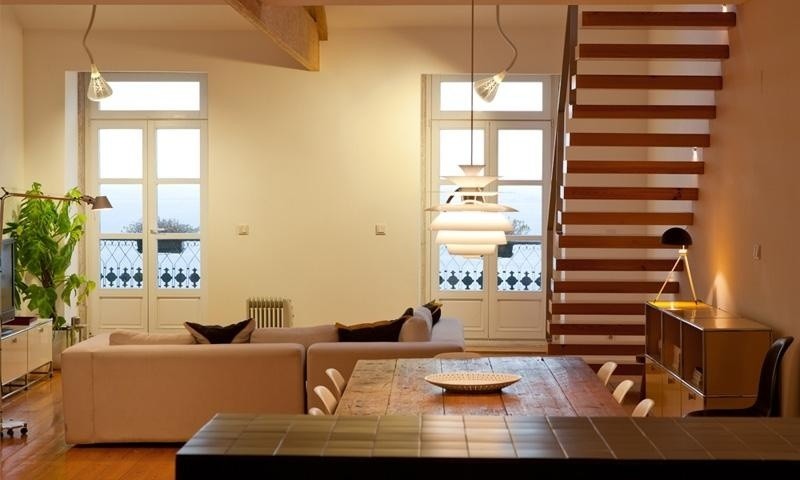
<box><xmin>425</xmin><ymin>372</ymin><xmax>521</xmax><ymax>393</ymax></box>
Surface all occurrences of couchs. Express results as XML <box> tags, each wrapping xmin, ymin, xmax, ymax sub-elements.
<box><xmin>58</xmin><ymin>301</ymin><xmax>464</xmax><ymax>444</ymax></box>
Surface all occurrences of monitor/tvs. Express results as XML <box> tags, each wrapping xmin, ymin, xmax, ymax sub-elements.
<box><xmin>0</xmin><ymin>238</ymin><xmax>17</xmax><ymax>336</ymax></box>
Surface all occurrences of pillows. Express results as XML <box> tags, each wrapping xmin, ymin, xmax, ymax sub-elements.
<box><xmin>184</xmin><ymin>318</ymin><xmax>255</xmax><ymax>343</ymax></box>
<box><xmin>250</xmin><ymin>325</ymin><xmax>341</xmax><ymax>346</ymax></box>
<box><xmin>425</xmin><ymin>301</ymin><xmax>441</xmax><ymax>325</ymax></box>
<box><xmin>399</xmin><ymin>306</ymin><xmax>432</xmax><ymax>342</ymax></box>
<box><xmin>335</xmin><ymin>308</ymin><xmax>413</xmax><ymax>342</ymax></box>
<box><xmin>109</xmin><ymin>328</ymin><xmax>196</xmax><ymax>344</ymax></box>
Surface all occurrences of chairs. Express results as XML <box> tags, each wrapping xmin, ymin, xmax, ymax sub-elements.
<box><xmin>687</xmin><ymin>335</ymin><xmax>793</xmax><ymax>415</ymax></box>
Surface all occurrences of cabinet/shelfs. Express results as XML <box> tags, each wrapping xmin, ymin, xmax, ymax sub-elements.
<box><xmin>1</xmin><ymin>316</ymin><xmax>55</xmax><ymax>400</ymax></box>
<box><xmin>644</xmin><ymin>299</ymin><xmax>772</xmax><ymax>417</ymax></box>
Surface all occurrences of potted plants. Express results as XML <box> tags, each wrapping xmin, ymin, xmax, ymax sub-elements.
<box><xmin>1</xmin><ymin>183</ymin><xmax>95</xmax><ymax>369</ymax></box>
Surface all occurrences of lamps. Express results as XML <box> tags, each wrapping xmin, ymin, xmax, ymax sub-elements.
<box><xmin>657</xmin><ymin>226</ymin><xmax>699</xmax><ymax>300</ymax></box>
<box><xmin>423</xmin><ymin>6</ymin><xmax>518</xmax><ymax>257</ymax></box>
<box><xmin>81</xmin><ymin>4</ymin><xmax>112</xmax><ymax>102</ymax></box>
<box><xmin>475</xmin><ymin>5</ymin><xmax>520</xmax><ymax>103</ymax></box>
<box><xmin>0</xmin><ymin>185</ymin><xmax>113</xmax><ymax>238</ymax></box>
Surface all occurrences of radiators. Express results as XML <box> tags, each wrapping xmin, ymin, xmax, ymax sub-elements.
<box><xmin>246</xmin><ymin>297</ymin><xmax>294</xmax><ymax>328</ymax></box>
